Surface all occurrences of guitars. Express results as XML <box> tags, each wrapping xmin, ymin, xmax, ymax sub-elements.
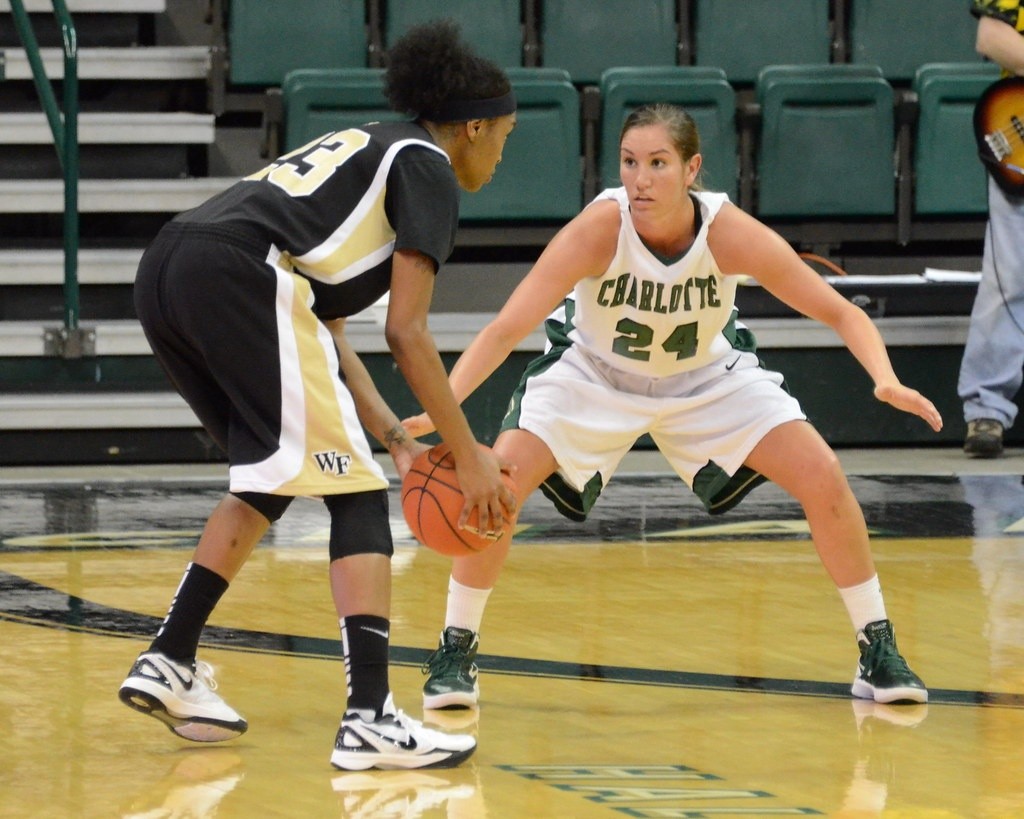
<box><xmin>971</xmin><ymin>70</ymin><xmax>1024</xmax><ymax>213</ymax></box>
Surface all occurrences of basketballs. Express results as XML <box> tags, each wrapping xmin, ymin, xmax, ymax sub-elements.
<box><xmin>399</xmin><ymin>437</ymin><xmax>521</xmax><ymax>558</ymax></box>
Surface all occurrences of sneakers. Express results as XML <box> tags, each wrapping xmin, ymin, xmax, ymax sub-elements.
<box><xmin>331</xmin><ymin>691</ymin><xmax>478</xmax><ymax>771</ymax></box>
<box><xmin>118</xmin><ymin>651</ymin><xmax>248</xmax><ymax>742</ymax></box>
<box><xmin>851</xmin><ymin>620</ymin><xmax>928</xmax><ymax>704</ymax></box>
<box><xmin>421</xmin><ymin>626</ymin><xmax>480</xmax><ymax>707</ymax></box>
<box><xmin>964</xmin><ymin>418</ymin><xmax>1003</xmax><ymax>457</ymax></box>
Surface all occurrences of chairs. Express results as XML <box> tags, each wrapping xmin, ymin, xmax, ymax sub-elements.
<box><xmin>203</xmin><ymin>0</ymin><xmax>1012</xmax><ymax>246</ymax></box>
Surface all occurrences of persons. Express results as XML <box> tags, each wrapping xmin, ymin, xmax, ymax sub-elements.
<box><xmin>958</xmin><ymin>0</ymin><xmax>1023</xmax><ymax>458</ymax></box>
<box><xmin>391</xmin><ymin>97</ymin><xmax>945</xmax><ymax>711</ymax></box>
<box><xmin>117</xmin><ymin>18</ymin><xmax>519</xmax><ymax>774</ymax></box>
